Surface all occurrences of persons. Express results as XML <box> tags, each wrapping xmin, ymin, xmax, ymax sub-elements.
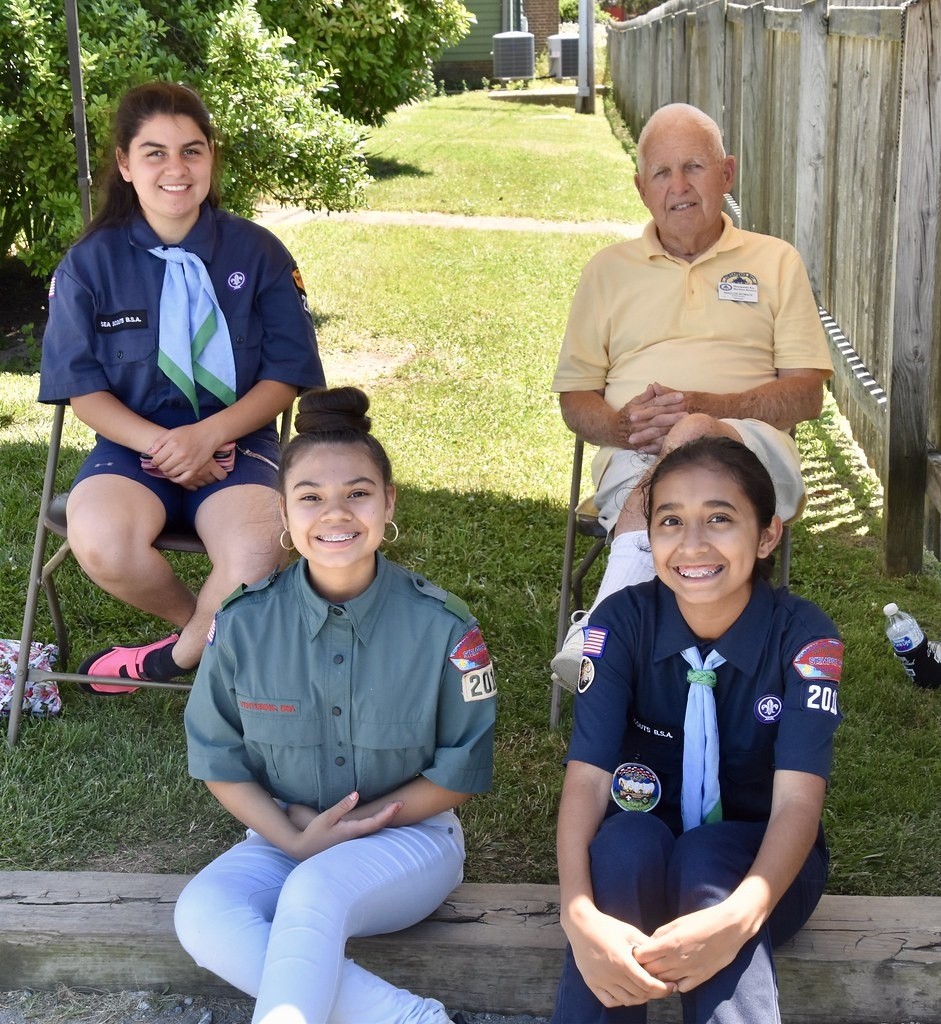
<box><xmin>173</xmin><ymin>386</ymin><xmax>498</xmax><ymax>1024</ymax></box>
<box><xmin>548</xmin><ymin>437</ymin><xmax>847</xmax><ymax>1024</ymax></box>
<box><xmin>36</xmin><ymin>83</ymin><xmax>326</xmax><ymax>697</ymax></box>
<box><xmin>551</xmin><ymin>102</ymin><xmax>836</xmax><ymax>695</ymax></box>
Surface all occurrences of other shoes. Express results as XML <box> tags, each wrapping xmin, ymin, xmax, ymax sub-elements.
<box><xmin>549</xmin><ymin>610</ymin><xmax>590</xmax><ymax>693</ymax></box>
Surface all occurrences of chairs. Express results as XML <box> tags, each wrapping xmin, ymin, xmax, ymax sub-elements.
<box><xmin>551</xmin><ymin>435</ymin><xmax>799</xmax><ymax>731</ymax></box>
<box><xmin>7</xmin><ymin>404</ymin><xmax>296</xmax><ymax>749</ymax></box>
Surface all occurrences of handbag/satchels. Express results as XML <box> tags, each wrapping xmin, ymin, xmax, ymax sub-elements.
<box><xmin>0</xmin><ymin>638</ymin><xmax>61</xmax><ymax>713</ymax></box>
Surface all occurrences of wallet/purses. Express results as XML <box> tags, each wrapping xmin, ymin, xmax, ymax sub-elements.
<box><xmin>139</xmin><ymin>440</ymin><xmax>238</xmax><ymax>477</ymax></box>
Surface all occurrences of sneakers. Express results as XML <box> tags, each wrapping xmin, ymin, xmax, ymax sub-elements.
<box><xmin>76</xmin><ymin>630</ymin><xmax>185</xmax><ymax>696</ymax></box>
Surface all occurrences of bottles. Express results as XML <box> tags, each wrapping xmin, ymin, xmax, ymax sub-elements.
<box><xmin>883</xmin><ymin>602</ymin><xmax>941</xmax><ymax>690</ymax></box>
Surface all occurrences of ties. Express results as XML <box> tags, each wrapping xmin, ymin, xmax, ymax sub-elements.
<box><xmin>149</xmin><ymin>244</ymin><xmax>239</xmax><ymax>419</ymax></box>
<box><xmin>678</xmin><ymin>647</ymin><xmax>726</xmax><ymax>832</ymax></box>
